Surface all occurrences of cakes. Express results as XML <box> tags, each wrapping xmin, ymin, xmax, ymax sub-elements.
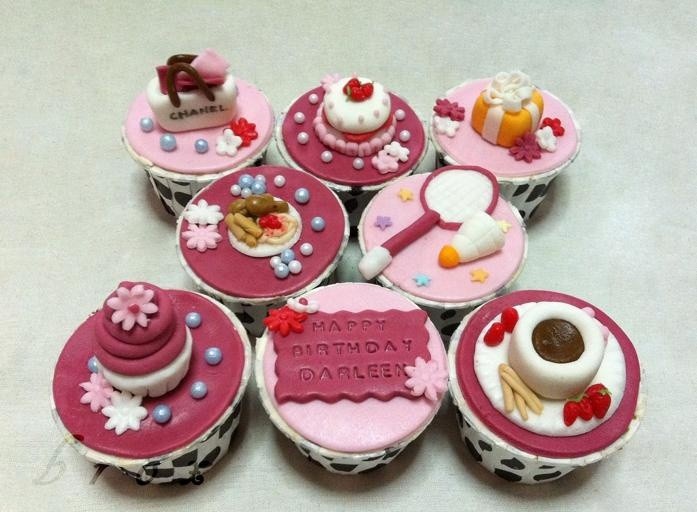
<box><xmin>53</xmin><ymin>54</ymin><xmax>640</xmax><ymax>486</ymax></box>
